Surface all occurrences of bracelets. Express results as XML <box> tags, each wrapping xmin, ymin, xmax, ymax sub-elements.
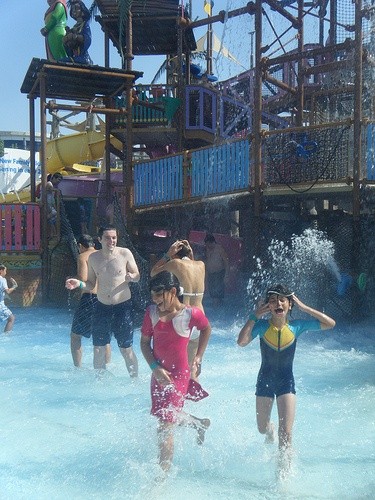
<box><xmin>163</xmin><ymin>253</ymin><xmax>172</xmax><ymax>263</ymax></box>
<box><xmin>249</xmin><ymin>313</ymin><xmax>258</xmax><ymax>322</ymax></box>
<box><xmin>79</xmin><ymin>281</ymin><xmax>82</xmax><ymax>289</ymax></box>
<box><xmin>149</xmin><ymin>360</ymin><xmax>159</xmax><ymax>370</ymax></box>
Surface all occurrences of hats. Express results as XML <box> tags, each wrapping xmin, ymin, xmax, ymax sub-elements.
<box><xmin>267</xmin><ymin>285</ymin><xmax>294</xmax><ymax>297</ymax></box>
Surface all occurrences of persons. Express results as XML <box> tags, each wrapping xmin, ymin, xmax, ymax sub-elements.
<box><xmin>141</xmin><ymin>270</ymin><xmax>211</xmax><ymax>485</ymax></box>
<box><xmin>77</xmin><ymin>197</ymin><xmax>89</xmax><ymax>234</ymax></box>
<box><xmin>0</xmin><ymin>264</ymin><xmax>18</xmax><ymax>332</ymax></box>
<box><xmin>150</xmin><ymin>239</ymin><xmax>205</xmax><ymax>383</ymax></box>
<box><xmin>237</xmin><ymin>284</ymin><xmax>336</xmax><ymax>485</ymax></box>
<box><xmin>40</xmin><ymin>172</ymin><xmax>64</xmax><ymax>245</ymax></box>
<box><xmin>65</xmin><ymin>225</ymin><xmax>140</xmax><ymax>378</ymax></box>
<box><xmin>62</xmin><ymin>0</ymin><xmax>93</xmax><ymax>64</ymax></box>
<box><xmin>105</xmin><ymin>195</ymin><xmax>120</xmax><ymax>225</ymax></box>
<box><xmin>204</xmin><ymin>234</ymin><xmax>229</xmax><ymax>305</ymax></box>
<box><xmin>40</xmin><ymin>0</ymin><xmax>68</xmax><ymax>60</ymax></box>
<box><xmin>70</xmin><ymin>234</ymin><xmax>112</xmax><ymax>368</ymax></box>
